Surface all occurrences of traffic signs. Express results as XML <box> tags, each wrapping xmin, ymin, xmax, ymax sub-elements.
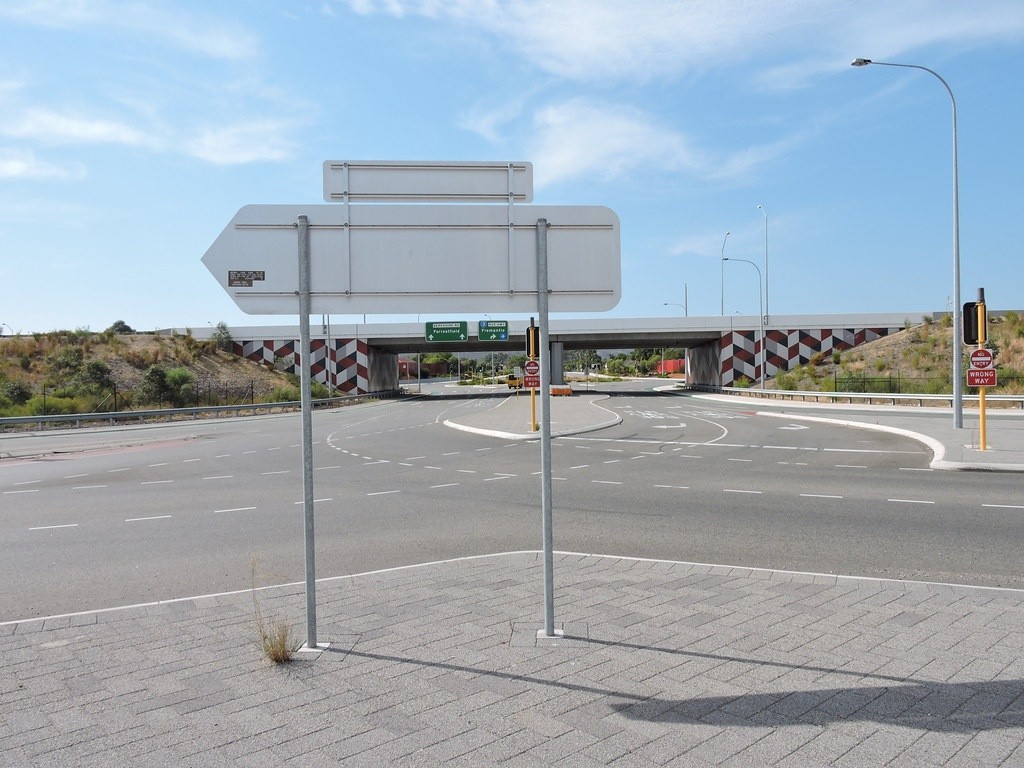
<box><xmin>478</xmin><ymin>320</ymin><xmax>509</xmax><ymax>341</ymax></box>
<box><xmin>425</xmin><ymin>321</ymin><xmax>468</xmax><ymax>342</ymax></box>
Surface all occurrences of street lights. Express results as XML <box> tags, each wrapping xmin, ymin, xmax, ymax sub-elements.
<box><xmin>850</xmin><ymin>57</ymin><xmax>963</xmax><ymax>429</ymax></box>
<box><xmin>720</xmin><ymin>232</ymin><xmax>731</xmax><ymax>315</ymax></box>
<box><xmin>758</xmin><ymin>204</ymin><xmax>769</xmax><ymax>325</ymax></box>
<box><xmin>665</xmin><ymin>302</ymin><xmax>688</xmax><ymax>318</ymax></box>
<box><xmin>722</xmin><ymin>257</ymin><xmax>765</xmax><ymax>389</ymax></box>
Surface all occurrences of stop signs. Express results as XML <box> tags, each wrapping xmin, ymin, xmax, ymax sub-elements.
<box><xmin>970</xmin><ymin>349</ymin><xmax>992</xmax><ymax>369</ymax></box>
<box><xmin>525</xmin><ymin>361</ymin><xmax>539</xmax><ymax>374</ymax></box>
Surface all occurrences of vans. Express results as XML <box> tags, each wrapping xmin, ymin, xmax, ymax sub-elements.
<box><xmin>508</xmin><ymin>373</ymin><xmax>523</xmax><ymax>389</ymax></box>
